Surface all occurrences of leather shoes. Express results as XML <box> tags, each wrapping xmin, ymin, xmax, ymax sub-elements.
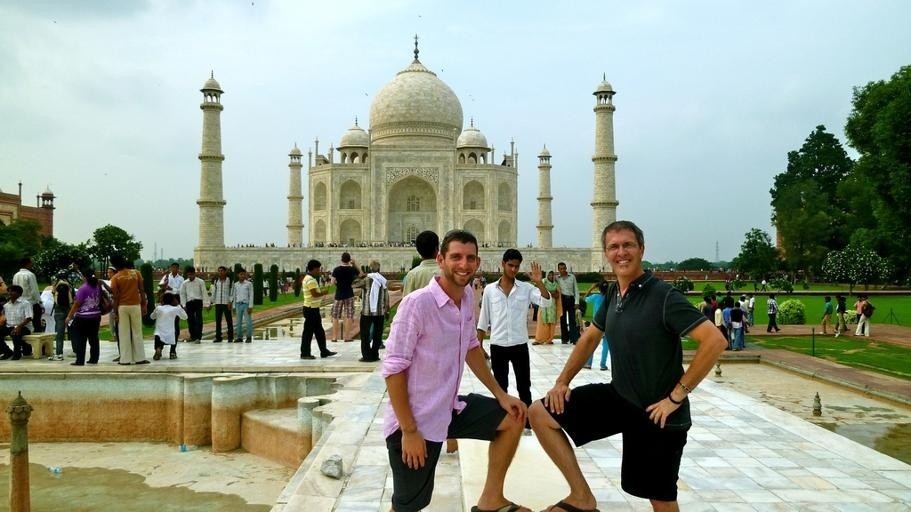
<box><xmin>301</xmin><ymin>354</ymin><xmax>316</xmax><ymax>359</ymax></box>
<box><xmin>321</xmin><ymin>351</ymin><xmax>337</xmax><ymax>358</ymax></box>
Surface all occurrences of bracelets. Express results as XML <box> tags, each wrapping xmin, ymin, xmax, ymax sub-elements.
<box><xmin>669</xmin><ymin>394</ymin><xmax>681</xmax><ymax>406</ymax></box>
<box><xmin>678</xmin><ymin>380</ymin><xmax>692</xmax><ymax>395</ymax></box>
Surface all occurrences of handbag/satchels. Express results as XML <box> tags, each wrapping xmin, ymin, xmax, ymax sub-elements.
<box><xmin>100</xmin><ymin>294</ymin><xmax>114</xmax><ymax>315</ymax></box>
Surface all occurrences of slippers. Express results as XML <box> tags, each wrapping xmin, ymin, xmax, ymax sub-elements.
<box><xmin>472</xmin><ymin>502</ymin><xmax>532</xmax><ymax>512</ymax></box>
<box><xmin>540</xmin><ymin>501</ymin><xmax>600</xmax><ymax>512</ymax></box>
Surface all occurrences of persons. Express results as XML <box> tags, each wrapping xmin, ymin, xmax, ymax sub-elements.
<box><xmin>404</xmin><ymin>231</ymin><xmax>442</xmax><ymax>294</ymax></box>
<box><xmin>229</xmin><ymin>239</ymin><xmax>519</xmax><ymax>249</ymax></box>
<box><xmin>471</xmin><ymin>274</ymin><xmax>492</xmax><ymax>311</ymax></box>
<box><xmin>532</xmin><ymin>269</ymin><xmax>563</xmax><ymax>345</ymax></box>
<box><xmin>263</xmin><ymin>279</ymin><xmax>286</xmax><ymax>296</ymax></box>
<box><xmin>150</xmin><ymin>264</ymin><xmax>255</xmax><ymax>360</ymax></box>
<box><xmin>725</xmin><ymin>277</ymin><xmax>730</xmax><ymax>290</ymax></box>
<box><xmin>380</xmin><ymin>230</ymin><xmax>535</xmax><ymax>512</ymax></box>
<box><xmin>697</xmin><ymin>292</ymin><xmax>755</xmax><ymax>351</ymax></box>
<box><xmin>541</xmin><ymin>262</ymin><xmax>580</xmax><ymax>345</ymax></box>
<box><xmin>761</xmin><ymin>279</ymin><xmax>767</xmax><ymax>291</ymax></box>
<box><xmin>766</xmin><ymin>293</ymin><xmax>781</xmax><ymax>333</ymax></box>
<box><xmin>477</xmin><ymin>249</ymin><xmax>552</xmax><ymax>437</ymax></box>
<box><xmin>582</xmin><ymin>280</ymin><xmax>609</xmax><ymax>371</ymax></box>
<box><xmin>1</xmin><ymin>253</ymin><xmax>147</xmax><ymax>366</ymax></box>
<box><xmin>330</xmin><ymin>253</ymin><xmax>359</xmax><ymax>342</ymax></box>
<box><xmin>359</xmin><ymin>261</ymin><xmax>388</xmax><ymax>362</ymax></box>
<box><xmin>821</xmin><ymin>297</ymin><xmax>837</xmax><ymax>334</ymax></box>
<box><xmin>321</xmin><ymin>272</ymin><xmax>332</xmax><ymax>286</ymax></box>
<box><xmin>729</xmin><ymin>278</ymin><xmax>735</xmax><ymax>291</ymax></box>
<box><xmin>753</xmin><ymin>279</ymin><xmax>760</xmax><ymax>291</ymax></box>
<box><xmin>834</xmin><ymin>292</ymin><xmax>875</xmax><ymax>337</ymax></box>
<box><xmin>300</xmin><ymin>259</ymin><xmax>337</xmax><ymax>359</ymax></box>
<box><xmin>528</xmin><ymin>221</ymin><xmax>728</xmax><ymax>512</ymax></box>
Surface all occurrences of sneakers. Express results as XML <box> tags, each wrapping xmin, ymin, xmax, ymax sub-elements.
<box><xmin>0</xmin><ymin>347</ymin><xmax>177</xmax><ymax>366</ymax></box>
<box><xmin>182</xmin><ymin>337</ymin><xmax>252</xmax><ymax>343</ymax></box>
